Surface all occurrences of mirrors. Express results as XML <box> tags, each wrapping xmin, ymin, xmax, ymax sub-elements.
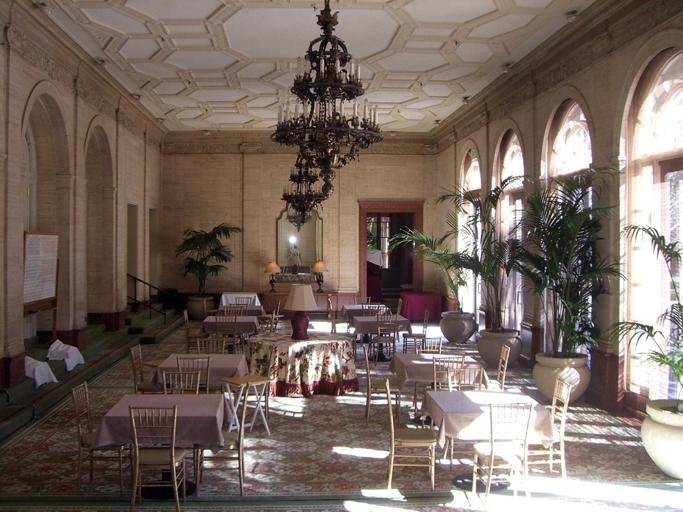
<box><xmin>275</xmin><ymin>204</ymin><xmax>324</xmax><ymax>273</ymax></box>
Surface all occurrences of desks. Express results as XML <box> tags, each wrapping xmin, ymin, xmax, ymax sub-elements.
<box><xmin>399</xmin><ymin>290</ymin><xmax>441</xmax><ymax>321</ymax></box>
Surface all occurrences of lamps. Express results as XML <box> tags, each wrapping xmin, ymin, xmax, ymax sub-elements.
<box><xmin>287</xmin><ymin>206</ymin><xmax>313</xmax><ymax>232</ymax></box>
<box><xmin>288</xmin><ymin>255</ymin><xmax>301</xmax><ymax>274</ymax></box>
<box><xmin>263</xmin><ymin>261</ymin><xmax>282</xmax><ymax>293</ymax></box>
<box><xmin>311</xmin><ymin>260</ymin><xmax>328</xmax><ymax>293</ymax></box>
<box><xmin>271</xmin><ymin>1</ymin><xmax>385</xmax><ymax>224</ymax></box>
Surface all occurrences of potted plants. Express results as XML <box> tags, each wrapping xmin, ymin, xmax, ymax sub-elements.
<box><xmin>174</xmin><ymin>221</ymin><xmax>243</xmax><ymax>320</ymax></box>
<box><xmin>386</xmin><ymin>226</ymin><xmax>481</xmax><ymax>343</ymax></box>
<box><xmin>431</xmin><ymin>173</ymin><xmax>535</xmax><ymax>368</ymax></box>
<box><xmin>595</xmin><ymin>225</ymin><xmax>682</xmax><ymax>481</ymax></box>
<box><xmin>507</xmin><ymin>165</ymin><xmax>628</xmax><ymax>405</ymax></box>
